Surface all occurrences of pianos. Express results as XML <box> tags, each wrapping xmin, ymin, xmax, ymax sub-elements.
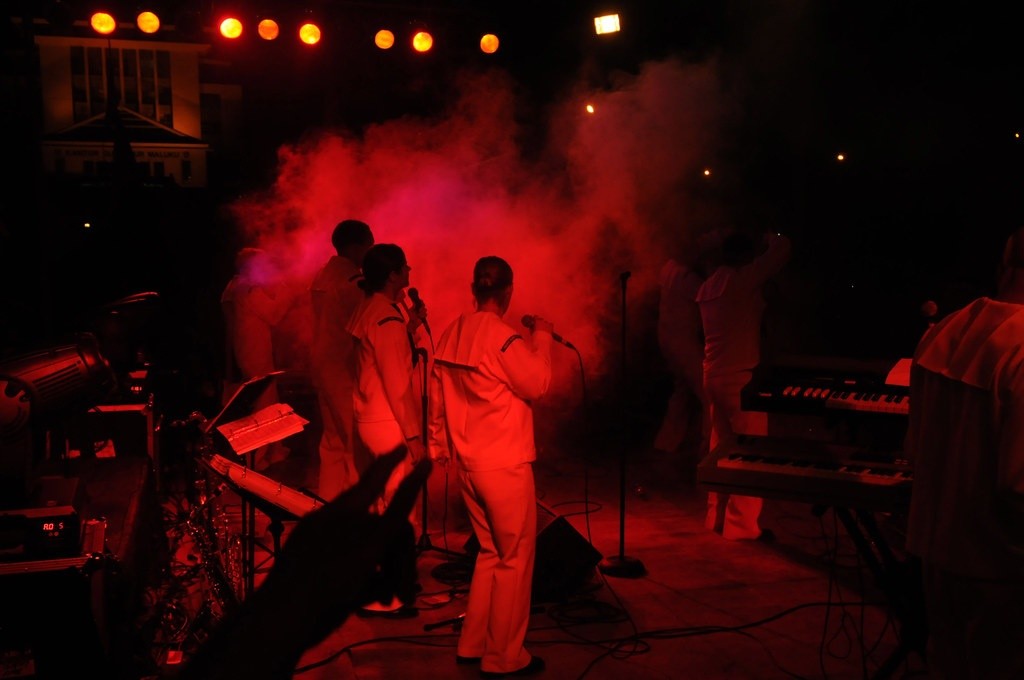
<box><xmin>757</xmin><ymin>383</ymin><xmax>910</xmax><ymax>424</ymax></box>
<box><xmin>696</xmin><ymin>435</ymin><xmax>906</xmax><ymax>512</ymax></box>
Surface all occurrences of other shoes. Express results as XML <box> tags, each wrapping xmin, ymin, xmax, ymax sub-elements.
<box><xmin>454</xmin><ymin>650</ymin><xmax>487</xmax><ymax>665</ymax></box>
<box><xmin>479</xmin><ymin>657</ymin><xmax>545</xmax><ymax>680</ymax></box>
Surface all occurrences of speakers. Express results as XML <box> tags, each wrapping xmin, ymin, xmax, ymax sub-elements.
<box><xmin>462</xmin><ymin>498</ymin><xmax>602</xmax><ymax>594</ymax></box>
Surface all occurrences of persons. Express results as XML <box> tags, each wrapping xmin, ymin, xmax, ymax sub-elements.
<box><xmin>652</xmin><ymin>226</ymin><xmax>790</xmax><ymax>544</ymax></box>
<box><xmin>426</xmin><ymin>255</ymin><xmax>552</xmax><ymax>680</ymax></box>
<box><xmin>903</xmin><ymin>231</ymin><xmax>1024</xmax><ymax>680</ymax></box>
<box><xmin>305</xmin><ymin>219</ymin><xmax>433</xmax><ymax>620</ymax></box>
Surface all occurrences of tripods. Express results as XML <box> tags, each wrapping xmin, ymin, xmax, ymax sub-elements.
<box><xmin>216</xmin><ymin>402</ymin><xmax>311</xmax><ymax>594</ymax></box>
<box><xmin>414</xmin><ymin>348</ymin><xmax>464</xmax><ymax>558</ymax></box>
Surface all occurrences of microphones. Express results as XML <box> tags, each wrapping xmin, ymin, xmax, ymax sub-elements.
<box><xmin>408</xmin><ymin>287</ymin><xmax>431</xmax><ymax>336</ymax></box>
<box><xmin>921</xmin><ymin>301</ymin><xmax>946</xmax><ymax>324</ymax></box>
<box><xmin>521</xmin><ymin>314</ymin><xmax>576</xmax><ymax>350</ymax></box>
